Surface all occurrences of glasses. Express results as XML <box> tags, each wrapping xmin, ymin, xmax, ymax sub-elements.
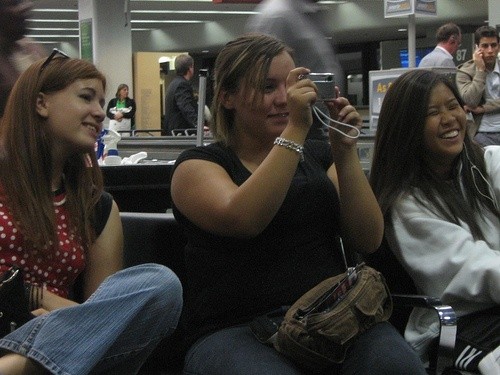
<box><xmin>38</xmin><ymin>47</ymin><xmax>72</xmax><ymax>77</ymax></box>
<box><xmin>450</xmin><ymin>36</ymin><xmax>463</xmax><ymax>48</ymax></box>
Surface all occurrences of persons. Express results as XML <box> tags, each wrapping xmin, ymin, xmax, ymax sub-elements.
<box><xmin>370</xmin><ymin>68</ymin><xmax>500</xmax><ymax>375</ymax></box>
<box><xmin>0</xmin><ymin>0</ymin><xmax>184</xmax><ymax>375</ymax></box>
<box><xmin>170</xmin><ymin>33</ymin><xmax>427</xmax><ymax>375</ymax></box>
<box><xmin>418</xmin><ymin>23</ymin><xmax>500</xmax><ymax>147</ymax></box>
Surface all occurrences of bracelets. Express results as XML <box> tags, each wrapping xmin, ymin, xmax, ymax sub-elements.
<box><xmin>274</xmin><ymin>137</ymin><xmax>304</xmax><ymax>162</ymax></box>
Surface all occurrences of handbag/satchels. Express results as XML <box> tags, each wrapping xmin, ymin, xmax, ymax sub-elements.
<box><xmin>247</xmin><ymin>261</ymin><xmax>393</xmax><ymax>375</ymax></box>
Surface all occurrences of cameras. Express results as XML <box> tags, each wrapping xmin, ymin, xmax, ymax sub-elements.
<box><xmin>298</xmin><ymin>72</ymin><xmax>336</xmax><ymax>102</ymax></box>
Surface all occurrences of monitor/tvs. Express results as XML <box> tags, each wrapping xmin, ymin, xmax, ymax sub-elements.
<box><xmin>398</xmin><ymin>46</ymin><xmax>436</xmax><ymax>68</ymax></box>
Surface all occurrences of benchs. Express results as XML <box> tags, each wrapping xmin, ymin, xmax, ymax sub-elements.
<box><xmin>104</xmin><ymin>192</ymin><xmax>475</xmax><ymax>375</ymax></box>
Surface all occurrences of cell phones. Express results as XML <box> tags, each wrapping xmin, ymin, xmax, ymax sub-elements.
<box><xmin>475</xmin><ymin>44</ymin><xmax>480</xmax><ymax>52</ymax></box>
